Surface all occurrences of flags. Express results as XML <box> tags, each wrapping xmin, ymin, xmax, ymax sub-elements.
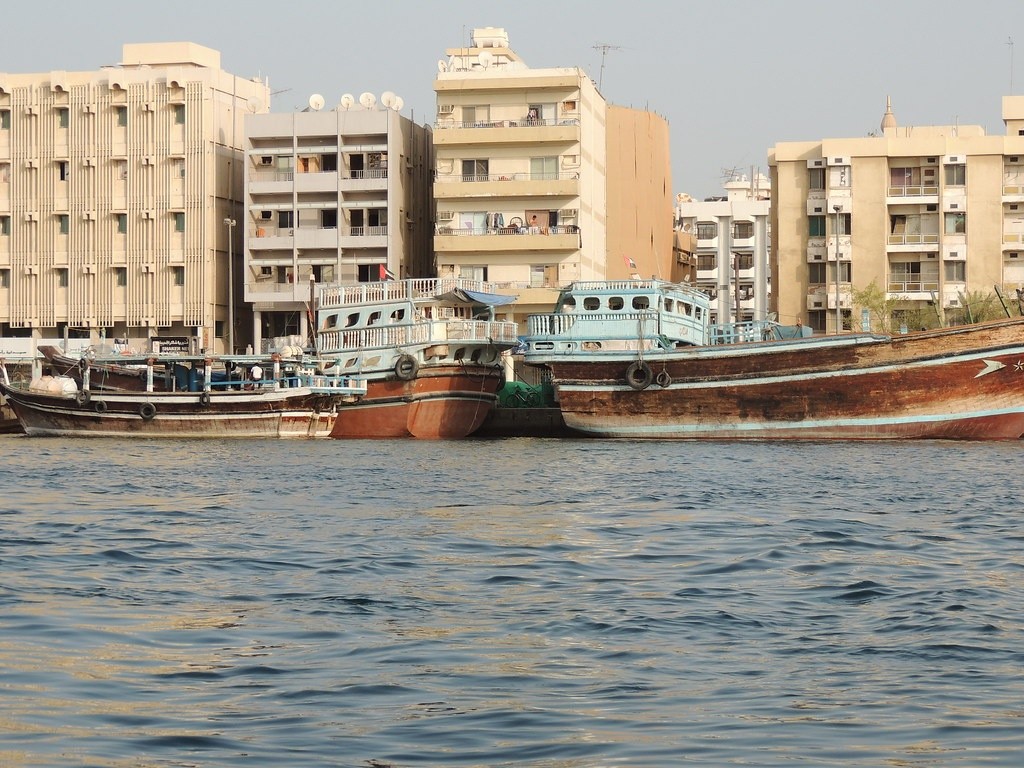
<box><xmin>379</xmin><ymin>264</ymin><xmax>394</xmax><ymax>280</ymax></box>
<box><xmin>624</xmin><ymin>255</ymin><xmax>636</xmax><ymax>269</ymax></box>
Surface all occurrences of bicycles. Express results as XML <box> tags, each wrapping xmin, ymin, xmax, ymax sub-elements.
<box><xmin>506</xmin><ymin>383</ymin><xmax>545</xmax><ymax>406</ymax></box>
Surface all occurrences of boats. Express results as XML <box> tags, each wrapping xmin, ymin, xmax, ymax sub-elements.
<box><xmin>1</xmin><ymin>277</ymin><xmax>519</xmax><ymax>441</ymax></box>
<box><xmin>519</xmin><ymin>275</ymin><xmax>1024</xmax><ymax>445</ymax></box>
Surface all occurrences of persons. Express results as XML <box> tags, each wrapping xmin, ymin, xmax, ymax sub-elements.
<box><xmin>287</xmin><ymin>273</ymin><xmax>294</xmax><ymax>282</ymax></box>
<box><xmin>529</xmin><ymin>215</ymin><xmax>537</xmax><ymax>226</ymax></box>
<box><xmin>528</xmin><ymin>109</ymin><xmax>536</xmax><ymax>119</ymax></box>
<box><xmin>250</xmin><ymin>363</ymin><xmax>263</xmax><ymax>389</ymax></box>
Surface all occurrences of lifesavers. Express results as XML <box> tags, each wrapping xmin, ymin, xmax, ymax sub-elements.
<box><xmin>656</xmin><ymin>370</ymin><xmax>672</xmax><ymax>387</ymax></box>
<box><xmin>626</xmin><ymin>361</ymin><xmax>654</xmax><ymax>390</ymax></box>
<box><xmin>140</xmin><ymin>402</ymin><xmax>157</xmax><ymax>421</ymax></box>
<box><xmin>394</xmin><ymin>354</ymin><xmax>419</xmax><ymax>382</ymax></box>
<box><xmin>95</xmin><ymin>400</ymin><xmax>108</xmax><ymax>413</ymax></box>
<box><xmin>75</xmin><ymin>389</ymin><xmax>91</xmax><ymax>406</ymax></box>
<box><xmin>199</xmin><ymin>393</ymin><xmax>210</xmax><ymax>406</ymax></box>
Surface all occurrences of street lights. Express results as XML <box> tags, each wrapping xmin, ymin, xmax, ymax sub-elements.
<box><xmin>223</xmin><ymin>219</ymin><xmax>237</xmax><ymax>356</ymax></box>
